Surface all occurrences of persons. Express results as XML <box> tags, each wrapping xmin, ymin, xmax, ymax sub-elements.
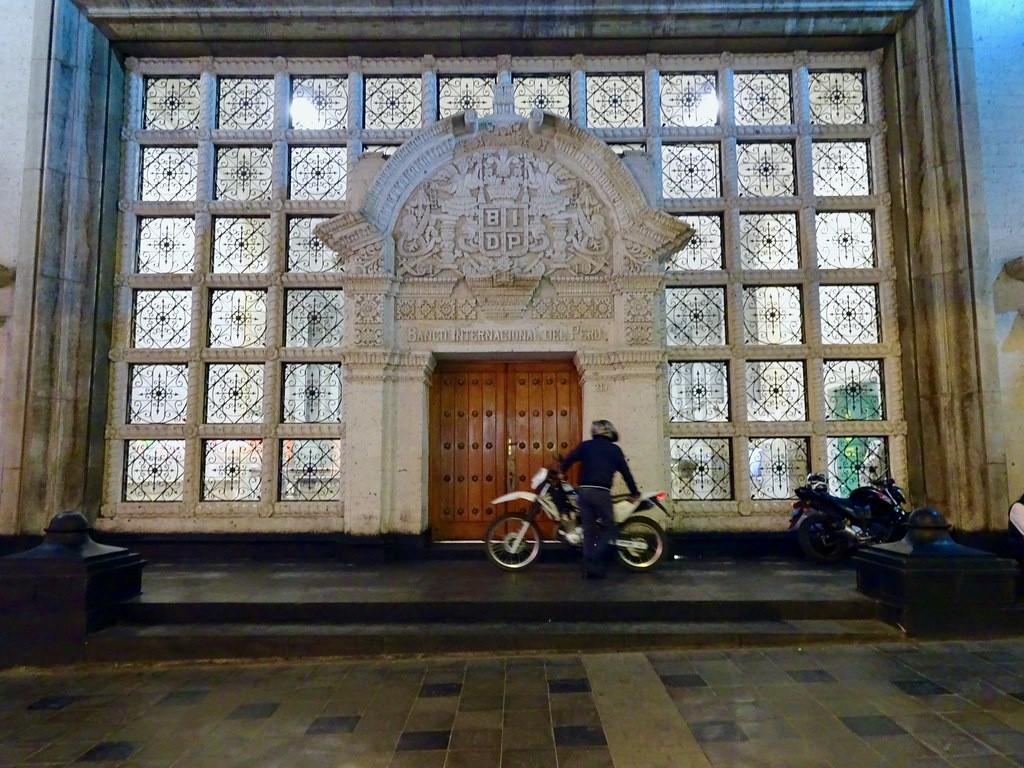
<box><xmin>1008</xmin><ymin>493</ymin><xmax>1024</xmax><ymax>567</ymax></box>
<box><xmin>558</xmin><ymin>420</ymin><xmax>640</xmax><ymax>580</ymax></box>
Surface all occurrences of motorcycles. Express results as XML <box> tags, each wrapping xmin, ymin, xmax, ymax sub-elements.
<box><xmin>482</xmin><ymin>450</ymin><xmax>674</xmax><ymax>573</ymax></box>
<box><xmin>787</xmin><ymin>467</ymin><xmax>912</xmax><ymax>566</ymax></box>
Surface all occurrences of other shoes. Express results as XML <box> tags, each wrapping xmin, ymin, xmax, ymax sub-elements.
<box><xmin>582</xmin><ymin>570</ymin><xmax>608</xmax><ymax>579</ymax></box>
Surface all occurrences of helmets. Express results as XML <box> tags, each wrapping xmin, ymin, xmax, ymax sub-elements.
<box><xmin>807</xmin><ymin>472</ymin><xmax>829</xmax><ymax>491</ymax></box>
<box><xmin>591</xmin><ymin>420</ymin><xmax>619</xmax><ymax>441</ymax></box>
